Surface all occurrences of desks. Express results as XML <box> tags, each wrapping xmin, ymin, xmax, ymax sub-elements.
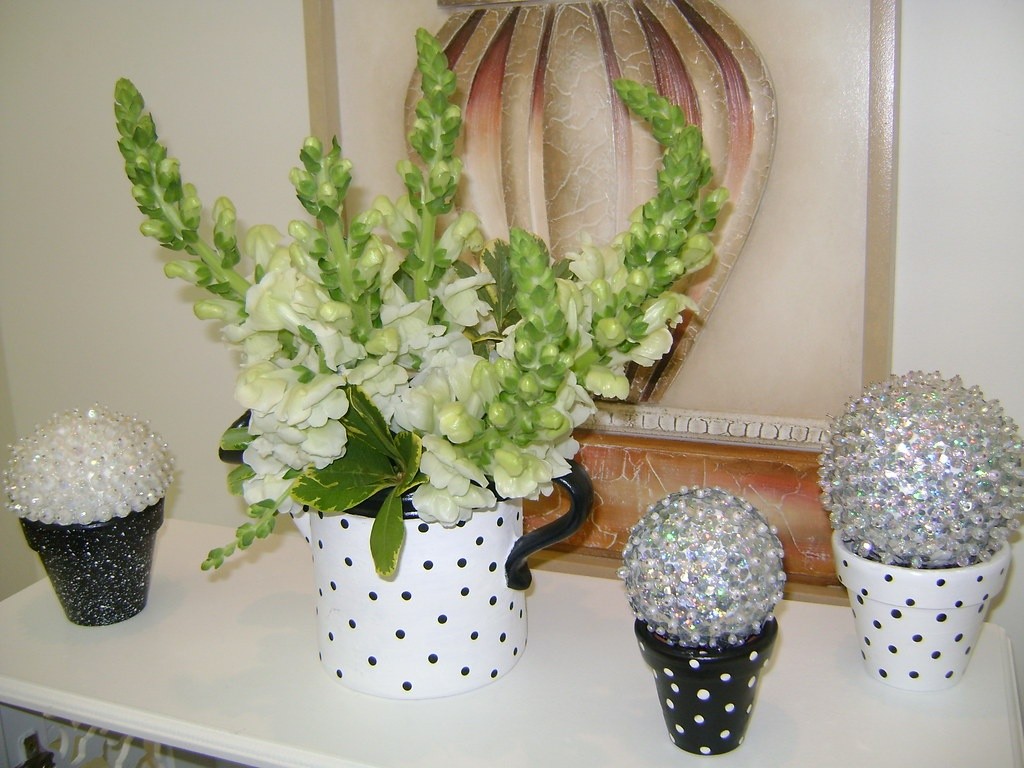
<box><xmin>0</xmin><ymin>558</ymin><xmax>1023</xmax><ymax>768</ymax></box>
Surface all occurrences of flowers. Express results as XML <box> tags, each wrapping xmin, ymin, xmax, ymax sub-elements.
<box><xmin>101</xmin><ymin>20</ymin><xmax>722</xmax><ymax>570</ymax></box>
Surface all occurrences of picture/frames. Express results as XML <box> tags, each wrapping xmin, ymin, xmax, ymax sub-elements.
<box><xmin>300</xmin><ymin>0</ymin><xmax>897</xmax><ymax>448</ymax></box>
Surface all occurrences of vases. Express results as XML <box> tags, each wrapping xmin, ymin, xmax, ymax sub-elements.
<box><xmin>15</xmin><ymin>504</ymin><xmax>167</xmax><ymax>624</ymax></box>
<box><xmin>633</xmin><ymin>619</ymin><xmax>776</xmax><ymax>758</ymax></box>
<box><xmin>828</xmin><ymin>535</ymin><xmax>1012</xmax><ymax>693</ymax></box>
<box><xmin>277</xmin><ymin>462</ymin><xmax>589</xmax><ymax>700</ymax></box>
